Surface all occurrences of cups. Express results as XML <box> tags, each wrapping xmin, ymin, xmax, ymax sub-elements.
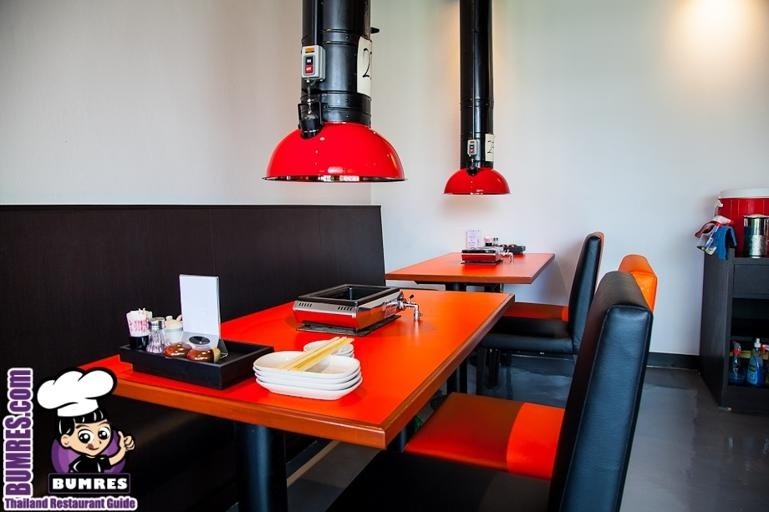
<box><xmin>466</xmin><ymin>225</ymin><xmax>481</xmax><ymax>251</ymax></box>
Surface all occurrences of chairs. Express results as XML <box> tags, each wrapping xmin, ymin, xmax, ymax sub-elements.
<box><xmin>326</xmin><ymin>270</ymin><xmax>656</xmax><ymax>511</ymax></box>
<box><xmin>477</xmin><ymin>231</ymin><xmax>605</xmax><ymax>392</ymax></box>
<box><xmin>401</xmin><ymin>254</ymin><xmax>659</xmax><ymax>479</ymax></box>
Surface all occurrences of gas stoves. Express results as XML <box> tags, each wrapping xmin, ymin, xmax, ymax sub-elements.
<box><xmin>462</xmin><ymin>247</ymin><xmax>504</xmax><ymax>263</ymax></box>
<box><xmin>292</xmin><ymin>283</ymin><xmax>405</xmax><ymax>337</ymax></box>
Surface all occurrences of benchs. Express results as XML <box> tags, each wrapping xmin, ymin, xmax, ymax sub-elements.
<box><xmin>0</xmin><ymin>204</ymin><xmax>383</xmax><ymax>511</ymax></box>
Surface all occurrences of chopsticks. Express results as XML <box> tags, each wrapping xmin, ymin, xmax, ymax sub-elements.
<box><xmin>126</xmin><ymin>308</ymin><xmax>153</xmax><ymax>336</ymax></box>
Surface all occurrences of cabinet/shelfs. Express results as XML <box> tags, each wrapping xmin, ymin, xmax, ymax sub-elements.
<box><xmin>700</xmin><ymin>224</ymin><xmax>769</xmax><ymax>415</ymax></box>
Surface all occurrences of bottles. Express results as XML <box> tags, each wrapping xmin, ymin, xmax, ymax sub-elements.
<box><xmin>484</xmin><ymin>237</ymin><xmax>499</xmax><ymax>248</ymax></box>
<box><xmin>128</xmin><ymin>334</ymin><xmax>148</xmax><ymax>349</ymax></box>
<box><xmin>146</xmin><ymin>318</ymin><xmax>215</xmax><ymax>362</ymax></box>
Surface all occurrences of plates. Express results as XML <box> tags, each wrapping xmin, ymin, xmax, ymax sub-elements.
<box><xmin>253</xmin><ymin>340</ymin><xmax>363</xmax><ymax>400</ymax></box>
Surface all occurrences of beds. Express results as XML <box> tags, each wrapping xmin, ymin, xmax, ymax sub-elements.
<box><xmin>78</xmin><ymin>289</ymin><xmax>515</xmax><ymax>511</ymax></box>
